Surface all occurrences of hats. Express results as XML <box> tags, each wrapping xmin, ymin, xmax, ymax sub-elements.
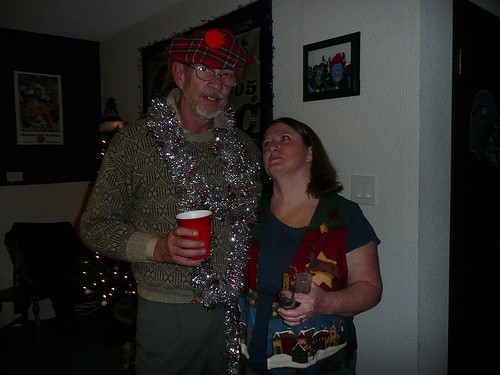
<box><xmin>171</xmin><ymin>28</ymin><xmax>254</xmax><ymax>70</ymax></box>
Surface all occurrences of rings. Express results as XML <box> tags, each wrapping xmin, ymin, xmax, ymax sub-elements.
<box><xmin>300</xmin><ymin>316</ymin><xmax>302</xmax><ymax>322</ymax></box>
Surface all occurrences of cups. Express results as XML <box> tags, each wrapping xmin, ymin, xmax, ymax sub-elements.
<box><xmin>176</xmin><ymin>210</ymin><xmax>213</xmax><ymax>261</ymax></box>
<box><xmin>296</xmin><ymin>273</ymin><xmax>311</xmax><ymax>293</ymax></box>
<box><xmin>278</xmin><ymin>288</ymin><xmax>295</xmax><ymax>309</ymax></box>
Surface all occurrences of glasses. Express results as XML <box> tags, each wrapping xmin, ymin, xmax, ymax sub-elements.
<box><xmin>178</xmin><ymin>61</ymin><xmax>236</xmax><ymax>87</ymax></box>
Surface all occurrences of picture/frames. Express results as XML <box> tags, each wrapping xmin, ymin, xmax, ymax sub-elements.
<box><xmin>302</xmin><ymin>31</ymin><xmax>361</xmax><ymax>103</ymax></box>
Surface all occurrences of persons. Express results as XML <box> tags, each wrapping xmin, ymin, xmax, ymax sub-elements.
<box><xmin>79</xmin><ymin>26</ymin><xmax>262</xmax><ymax>375</ymax></box>
<box><xmin>240</xmin><ymin>118</ymin><xmax>383</xmax><ymax>375</ymax></box>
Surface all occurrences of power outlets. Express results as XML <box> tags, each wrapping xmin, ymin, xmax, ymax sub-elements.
<box><xmin>6</xmin><ymin>172</ymin><xmax>24</xmax><ymax>182</ymax></box>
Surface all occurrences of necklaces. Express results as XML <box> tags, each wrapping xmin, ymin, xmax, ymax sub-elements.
<box><xmin>146</xmin><ymin>97</ymin><xmax>255</xmax><ymax>375</ymax></box>
